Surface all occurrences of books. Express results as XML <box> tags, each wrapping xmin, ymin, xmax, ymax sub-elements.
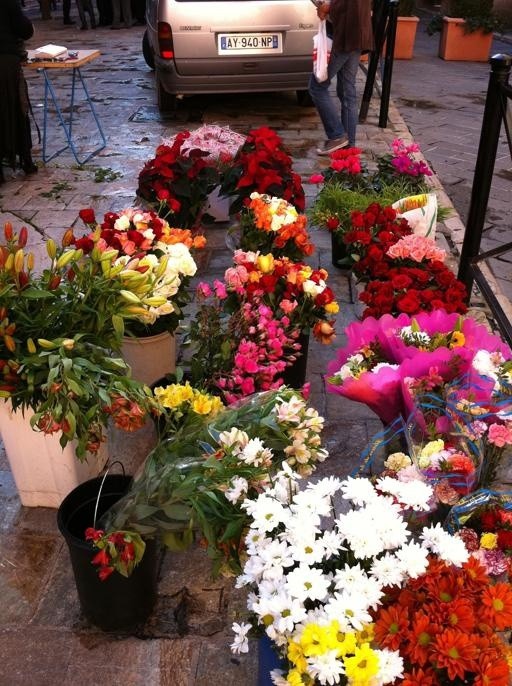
<box><xmin>35</xmin><ymin>44</ymin><xmax>67</xmax><ymax>59</ymax></box>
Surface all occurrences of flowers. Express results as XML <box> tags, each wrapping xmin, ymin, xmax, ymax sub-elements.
<box><xmin>3</xmin><ymin>127</ymin><xmax>512</xmax><ymax>684</ymax></box>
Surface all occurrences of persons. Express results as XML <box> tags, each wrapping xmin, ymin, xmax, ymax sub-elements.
<box><xmin>21</xmin><ymin>0</ymin><xmax>146</xmax><ymax>30</ymax></box>
<box><xmin>0</xmin><ymin>0</ymin><xmax>38</xmax><ymax>188</ymax></box>
<box><xmin>307</xmin><ymin>0</ymin><xmax>374</xmax><ymax>156</ymax></box>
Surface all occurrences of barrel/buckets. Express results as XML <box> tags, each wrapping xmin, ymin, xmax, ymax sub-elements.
<box><xmin>57</xmin><ymin>461</ymin><xmax>161</xmax><ymax>629</ymax></box>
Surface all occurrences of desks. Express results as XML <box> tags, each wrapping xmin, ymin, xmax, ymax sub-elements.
<box><xmin>23</xmin><ymin>50</ymin><xmax>108</xmax><ymax>164</ymax></box>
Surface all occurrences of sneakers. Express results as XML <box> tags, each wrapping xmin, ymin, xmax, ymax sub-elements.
<box><xmin>315</xmin><ymin>136</ymin><xmax>350</xmax><ymax>157</ymax></box>
<box><xmin>37</xmin><ymin>7</ymin><xmax>147</xmax><ymax>30</ymax></box>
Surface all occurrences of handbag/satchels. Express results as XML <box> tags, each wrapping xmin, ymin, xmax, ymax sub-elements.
<box><xmin>310</xmin><ymin>19</ymin><xmax>334</xmax><ymax>84</ymax></box>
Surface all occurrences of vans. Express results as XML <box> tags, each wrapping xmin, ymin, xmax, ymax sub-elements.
<box><xmin>141</xmin><ymin>0</ymin><xmax>322</xmax><ymax>111</ymax></box>
